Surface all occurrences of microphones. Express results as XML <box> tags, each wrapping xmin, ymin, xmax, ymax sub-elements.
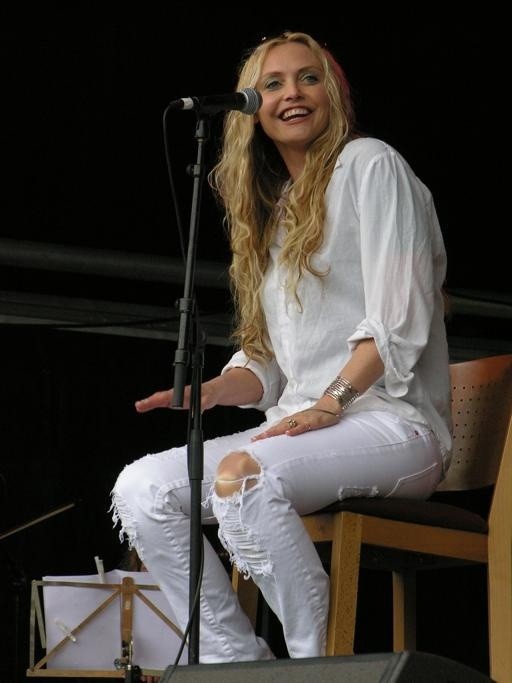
<box><xmin>178</xmin><ymin>87</ymin><xmax>263</xmax><ymax>116</ymax></box>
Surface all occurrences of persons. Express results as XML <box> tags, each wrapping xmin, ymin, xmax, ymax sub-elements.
<box><xmin>106</xmin><ymin>31</ymin><xmax>452</xmax><ymax>667</ymax></box>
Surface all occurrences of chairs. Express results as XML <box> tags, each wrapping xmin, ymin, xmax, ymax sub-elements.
<box><xmin>231</xmin><ymin>352</ymin><xmax>511</xmax><ymax>683</ymax></box>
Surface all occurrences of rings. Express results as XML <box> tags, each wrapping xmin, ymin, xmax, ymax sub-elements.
<box><xmin>305</xmin><ymin>421</ymin><xmax>312</xmax><ymax>430</ymax></box>
<box><xmin>286</xmin><ymin>419</ymin><xmax>297</xmax><ymax>427</ymax></box>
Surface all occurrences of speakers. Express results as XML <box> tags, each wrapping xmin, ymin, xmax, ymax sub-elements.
<box><xmin>161</xmin><ymin>651</ymin><xmax>499</xmax><ymax>683</ymax></box>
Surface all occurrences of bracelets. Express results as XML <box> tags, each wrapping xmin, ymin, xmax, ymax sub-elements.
<box><xmin>304</xmin><ymin>405</ymin><xmax>339</xmax><ymax>417</ymax></box>
<box><xmin>325</xmin><ymin>373</ymin><xmax>359</xmax><ymax>409</ymax></box>
